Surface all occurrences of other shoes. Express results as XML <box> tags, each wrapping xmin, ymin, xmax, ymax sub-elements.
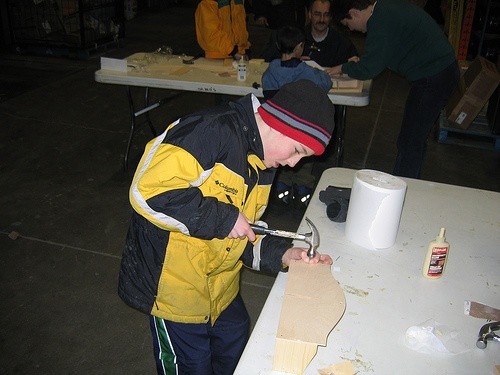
<box><xmin>265</xmin><ymin>182</ymin><xmax>292</xmax><ymax>218</ymax></box>
<box><xmin>292</xmin><ymin>182</ymin><xmax>315</xmax><ymax>218</ymax></box>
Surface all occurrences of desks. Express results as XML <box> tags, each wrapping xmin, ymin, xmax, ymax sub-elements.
<box><xmin>95</xmin><ymin>51</ymin><xmax>375</xmax><ymax>174</ymax></box>
<box><xmin>233</xmin><ymin>167</ymin><xmax>500</xmax><ymax>375</ymax></box>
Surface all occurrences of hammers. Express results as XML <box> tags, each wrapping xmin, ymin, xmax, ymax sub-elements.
<box><xmin>182</xmin><ymin>53</ymin><xmax>203</xmax><ymax>65</ymax></box>
<box><xmin>308</xmin><ymin>45</ymin><xmax>320</xmax><ymax>56</ymax></box>
<box><xmin>474</xmin><ymin>321</ymin><xmax>500</xmax><ymax>349</ymax></box>
<box><xmin>249</xmin><ymin>217</ymin><xmax>320</xmax><ymax>259</ymax></box>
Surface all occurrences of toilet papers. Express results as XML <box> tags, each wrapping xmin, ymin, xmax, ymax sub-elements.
<box><xmin>343</xmin><ymin>168</ymin><xmax>409</xmax><ymax>253</ymax></box>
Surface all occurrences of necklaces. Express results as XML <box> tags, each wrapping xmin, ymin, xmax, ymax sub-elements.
<box><xmin>369</xmin><ymin>1</ymin><xmax>377</xmax><ymax>17</ymax></box>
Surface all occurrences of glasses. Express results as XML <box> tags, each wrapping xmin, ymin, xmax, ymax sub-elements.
<box><xmin>310</xmin><ymin>10</ymin><xmax>331</xmax><ymax>18</ymax></box>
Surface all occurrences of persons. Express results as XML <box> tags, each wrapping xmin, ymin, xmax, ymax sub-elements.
<box><xmin>117</xmin><ymin>79</ymin><xmax>336</xmax><ymax>375</ymax></box>
<box><xmin>195</xmin><ymin>0</ymin><xmax>252</xmax><ymax>105</ymax></box>
<box><xmin>262</xmin><ymin>25</ymin><xmax>332</xmax><ymax>217</ymax></box>
<box><xmin>253</xmin><ymin>0</ymin><xmax>306</xmax><ymax>45</ymax></box>
<box><xmin>325</xmin><ymin>0</ymin><xmax>458</xmax><ymax>179</ymax></box>
<box><xmin>271</xmin><ymin>0</ymin><xmax>359</xmax><ymax>68</ymax></box>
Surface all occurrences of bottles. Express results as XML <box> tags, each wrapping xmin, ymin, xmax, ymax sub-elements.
<box><xmin>237</xmin><ymin>56</ymin><xmax>249</xmax><ymax>81</ymax></box>
<box><xmin>422</xmin><ymin>227</ymin><xmax>450</xmax><ymax>278</ymax></box>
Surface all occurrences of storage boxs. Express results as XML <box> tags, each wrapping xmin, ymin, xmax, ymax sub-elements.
<box><xmin>444</xmin><ymin>54</ymin><xmax>500</xmax><ymax>129</ymax></box>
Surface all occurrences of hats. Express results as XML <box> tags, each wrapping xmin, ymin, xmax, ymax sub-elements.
<box><xmin>258</xmin><ymin>79</ymin><xmax>335</xmax><ymax>155</ymax></box>
